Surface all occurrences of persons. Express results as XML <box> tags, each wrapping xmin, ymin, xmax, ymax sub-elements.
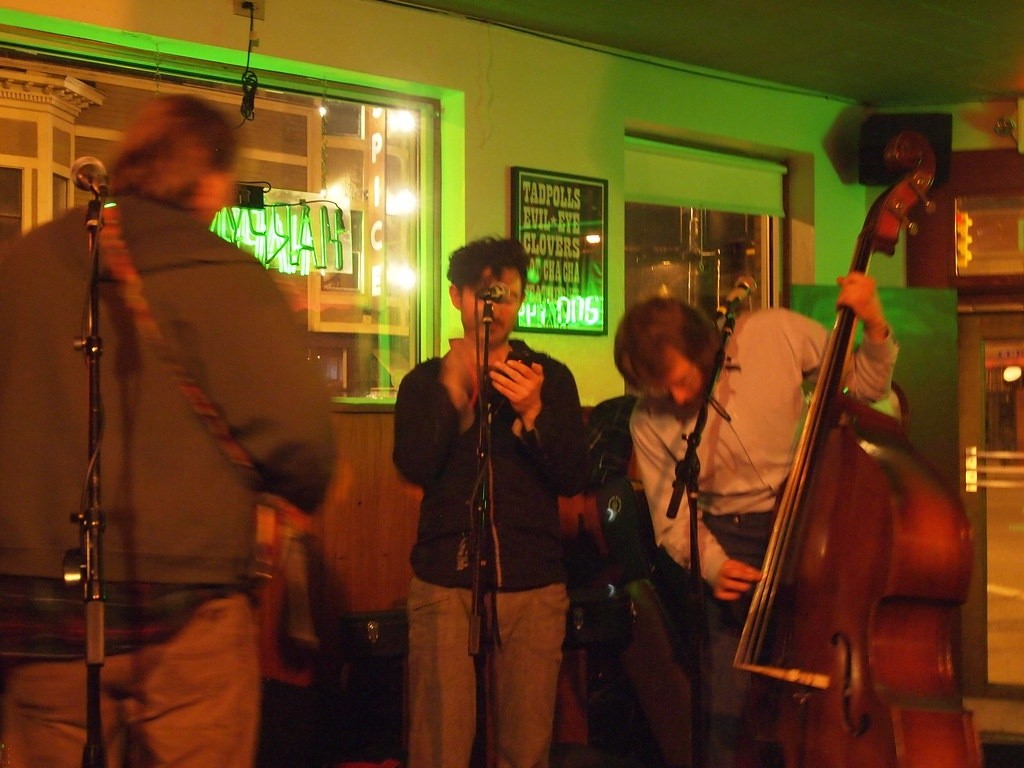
<box><xmin>611</xmin><ymin>271</ymin><xmax>903</xmax><ymax>766</ymax></box>
<box><xmin>390</xmin><ymin>236</ymin><xmax>589</xmax><ymax>767</ymax></box>
<box><xmin>0</xmin><ymin>96</ymin><xmax>338</xmax><ymax>768</ymax></box>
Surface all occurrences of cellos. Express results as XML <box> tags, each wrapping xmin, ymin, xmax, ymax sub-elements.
<box><xmin>732</xmin><ymin>128</ymin><xmax>1024</xmax><ymax>768</ymax></box>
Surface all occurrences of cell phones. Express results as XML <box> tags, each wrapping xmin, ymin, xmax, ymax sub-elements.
<box><xmin>500</xmin><ymin>351</ymin><xmax>533</xmax><ymax>381</ymax></box>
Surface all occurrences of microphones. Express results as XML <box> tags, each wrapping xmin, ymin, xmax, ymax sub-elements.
<box><xmin>480</xmin><ymin>281</ymin><xmax>510</xmax><ymax>303</ymax></box>
<box><xmin>713</xmin><ymin>276</ymin><xmax>756</xmax><ymax>320</ymax></box>
<box><xmin>71</xmin><ymin>157</ymin><xmax>109</xmax><ymax>196</ymax></box>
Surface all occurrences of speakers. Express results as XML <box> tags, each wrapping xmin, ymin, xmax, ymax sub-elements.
<box><xmin>858</xmin><ymin>112</ymin><xmax>952</xmax><ymax>185</ymax></box>
